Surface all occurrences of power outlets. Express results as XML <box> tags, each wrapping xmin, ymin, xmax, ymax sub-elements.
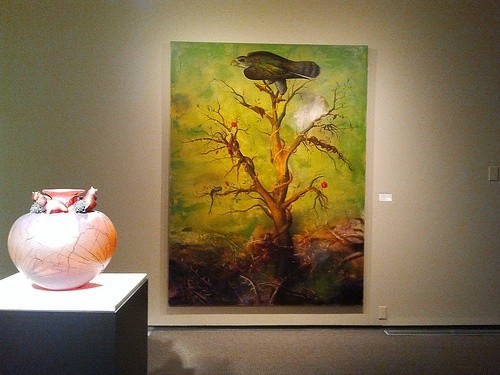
<box><xmin>488</xmin><ymin>166</ymin><xmax>498</xmax><ymax>181</ymax></box>
<box><xmin>379</xmin><ymin>306</ymin><xmax>387</xmax><ymax>320</ymax></box>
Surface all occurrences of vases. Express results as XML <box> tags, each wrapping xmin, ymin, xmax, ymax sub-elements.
<box><xmin>7</xmin><ymin>186</ymin><xmax>118</xmax><ymax>291</ymax></box>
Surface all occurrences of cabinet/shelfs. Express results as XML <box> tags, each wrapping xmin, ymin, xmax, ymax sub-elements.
<box><xmin>0</xmin><ymin>271</ymin><xmax>149</xmax><ymax>375</ymax></box>
